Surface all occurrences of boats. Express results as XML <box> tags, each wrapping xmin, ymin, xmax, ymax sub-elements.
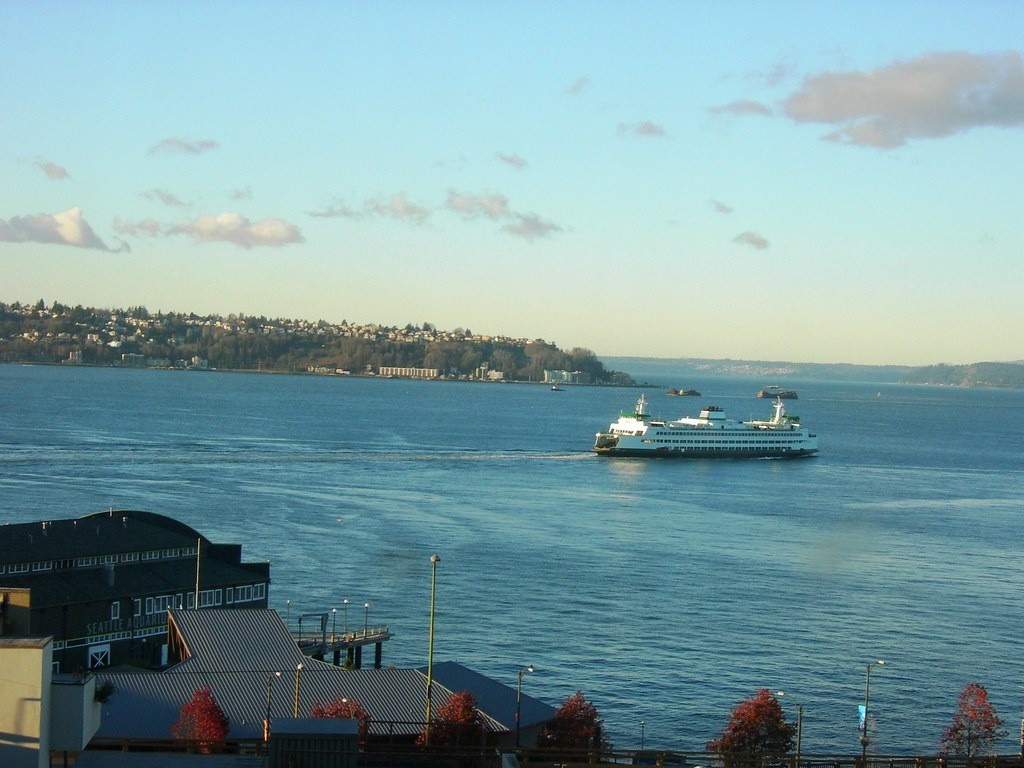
<box><xmin>551</xmin><ymin>384</ymin><xmax>560</xmax><ymax>391</ymax></box>
<box><xmin>590</xmin><ymin>392</ymin><xmax>820</xmax><ymax>460</ymax></box>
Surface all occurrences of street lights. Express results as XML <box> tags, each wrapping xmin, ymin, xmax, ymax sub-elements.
<box><xmin>862</xmin><ymin>658</ymin><xmax>887</xmax><ymax>758</ymax></box>
<box><xmin>331</xmin><ymin>608</ymin><xmax>336</xmax><ymax>643</ymax></box>
<box><xmin>342</xmin><ymin>599</ymin><xmax>349</xmax><ymax>638</ymax></box>
<box><xmin>294</xmin><ymin>662</ymin><xmax>305</xmax><ymax>718</ymax></box>
<box><xmin>424</xmin><ymin>554</ymin><xmax>442</xmax><ymax>746</ymax></box>
<box><xmin>363</xmin><ymin>601</ymin><xmax>370</xmax><ymax>639</ymax></box>
<box><xmin>260</xmin><ymin>702</ymin><xmax>263</xmax><ymax>703</ymax></box>
<box><xmin>262</xmin><ymin>669</ymin><xmax>283</xmax><ymax>734</ymax></box>
<box><xmin>514</xmin><ymin>663</ymin><xmax>535</xmax><ymax>747</ymax></box>
<box><xmin>286</xmin><ymin>600</ymin><xmax>290</xmax><ymax>630</ymax></box>
